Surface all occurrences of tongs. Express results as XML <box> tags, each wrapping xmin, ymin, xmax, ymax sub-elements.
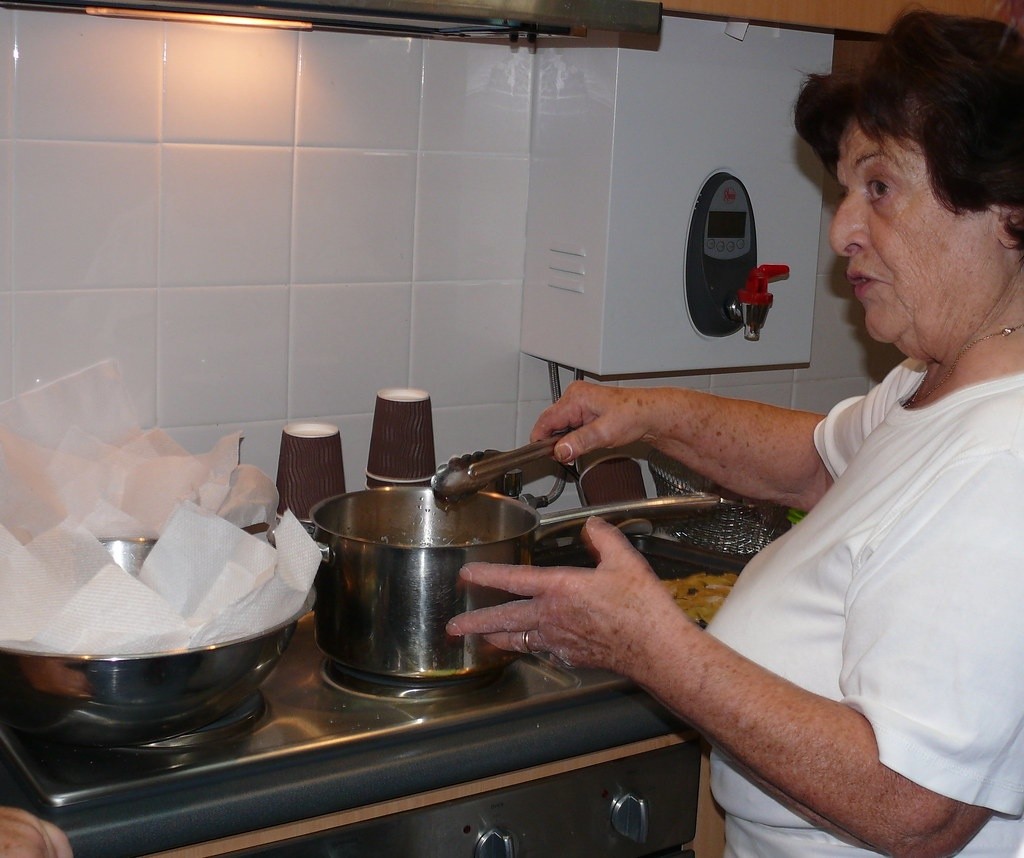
<box><xmin>432</xmin><ymin>432</ymin><xmax>570</xmax><ymax>496</ymax></box>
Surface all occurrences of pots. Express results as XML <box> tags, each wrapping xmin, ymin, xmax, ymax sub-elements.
<box><xmin>0</xmin><ymin>525</ymin><xmax>333</xmax><ymax>743</ymax></box>
<box><xmin>311</xmin><ymin>481</ymin><xmax>722</xmax><ymax>689</ymax></box>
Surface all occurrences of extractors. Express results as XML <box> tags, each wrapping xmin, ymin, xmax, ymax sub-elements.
<box><xmin>0</xmin><ymin>0</ymin><xmax>666</xmax><ymax>51</ymax></box>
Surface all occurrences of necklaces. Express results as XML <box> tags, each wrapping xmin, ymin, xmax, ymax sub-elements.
<box><xmin>902</xmin><ymin>324</ymin><xmax>1024</xmax><ymax>409</ymax></box>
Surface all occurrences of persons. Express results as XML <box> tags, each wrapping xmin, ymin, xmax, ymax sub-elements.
<box><xmin>446</xmin><ymin>16</ymin><xmax>1024</xmax><ymax>858</ymax></box>
<box><xmin>0</xmin><ymin>806</ymin><xmax>74</xmax><ymax>858</ymax></box>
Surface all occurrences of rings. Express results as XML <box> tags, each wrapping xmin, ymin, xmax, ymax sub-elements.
<box><xmin>522</xmin><ymin>631</ymin><xmax>541</xmax><ymax>653</ymax></box>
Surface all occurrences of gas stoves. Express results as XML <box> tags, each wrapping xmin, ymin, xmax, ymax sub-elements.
<box><xmin>0</xmin><ymin>610</ymin><xmax>707</xmax><ymax>858</ymax></box>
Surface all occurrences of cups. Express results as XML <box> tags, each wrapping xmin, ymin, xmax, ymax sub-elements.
<box><xmin>577</xmin><ymin>456</ymin><xmax>651</xmax><ymax>541</ymax></box>
<box><xmin>271</xmin><ymin>420</ymin><xmax>345</xmax><ymax>531</ymax></box>
<box><xmin>364</xmin><ymin>389</ymin><xmax>436</xmax><ymax>493</ymax></box>
